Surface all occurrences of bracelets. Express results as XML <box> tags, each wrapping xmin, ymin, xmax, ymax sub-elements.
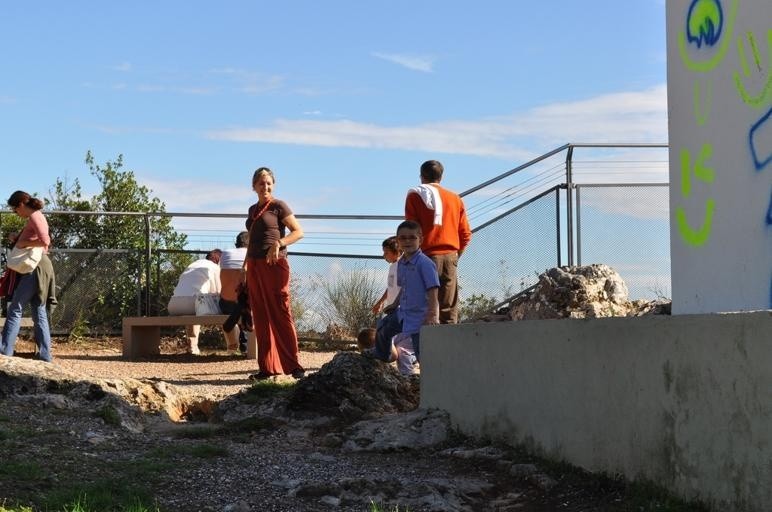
<box><xmin>278</xmin><ymin>239</ymin><xmax>285</xmax><ymax>247</ymax></box>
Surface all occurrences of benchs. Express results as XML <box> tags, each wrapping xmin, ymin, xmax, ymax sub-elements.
<box><xmin>122</xmin><ymin>315</ymin><xmax>258</xmax><ymax>360</ymax></box>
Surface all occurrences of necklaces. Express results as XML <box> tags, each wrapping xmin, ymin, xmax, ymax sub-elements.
<box><xmin>252</xmin><ymin>199</ymin><xmax>272</xmax><ymax>221</ymax></box>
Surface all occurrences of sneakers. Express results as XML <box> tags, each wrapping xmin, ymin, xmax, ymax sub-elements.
<box><xmin>186</xmin><ymin>347</ymin><xmax>200</xmax><ymax>355</ymax></box>
<box><xmin>249</xmin><ymin>370</ymin><xmax>305</xmax><ymax>380</ymax></box>
<box><xmin>226</xmin><ymin>345</ymin><xmax>239</xmax><ymax>355</ymax></box>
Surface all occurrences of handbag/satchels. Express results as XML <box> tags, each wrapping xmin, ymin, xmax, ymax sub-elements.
<box><xmin>6</xmin><ymin>244</ymin><xmax>44</xmax><ymax>275</ymax></box>
<box><xmin>195</xmin><ymin>292</ymin><xmax>222</xmax><ymax>317</ymax></box>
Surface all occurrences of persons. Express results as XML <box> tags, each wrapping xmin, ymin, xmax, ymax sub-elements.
<box><xmin>358</xmin><ymin>221</ymin><xmax>440</xmax><ymax>375</ymax></box>
<box><xmin>405</xmin><ymin>160</ymin><xmax>471</xmax><ymax>324</ymax></box>
<box><xmin>219</xmin><ymin>232</ymin><xmax>250</xmax><ymax>356</ymax></box>
<box><xmin>0</xmin><ymin>191</ymin><xmax>58</xmax><ymax>362</ymax></box>
<box><xmin>168</xmin><ymin>249</ymin><xmax>238</xmax><ymax>355</ymax></box>
<box><xmin>243</xmin><ymin>168</ymin><xmax>305</xmax><ymax>380</ymax></box>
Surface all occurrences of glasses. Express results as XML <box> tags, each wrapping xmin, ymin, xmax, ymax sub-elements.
<box><xmin>399</xmin><ymin>234</ymin><xmax>417</xmax><ymax>239</ymax></box>
